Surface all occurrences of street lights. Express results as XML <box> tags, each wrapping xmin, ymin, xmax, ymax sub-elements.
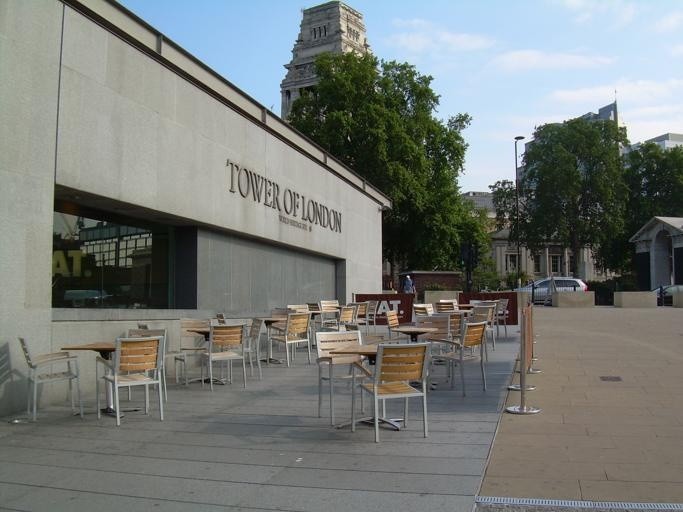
<box><xmin>513</xmin><ymin>135</ymin><xmax>527</xmax><ymax>288</ymax></box>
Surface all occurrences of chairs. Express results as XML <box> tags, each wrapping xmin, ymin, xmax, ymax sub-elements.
<box><xmin>200</xmin><ymin>324</ymin><xmax>246</xmax><ymax>391</ymax></box>
<box><xmin>224</xmin><ymin>317</ymin><xmax>263</xmax><ymax>382</ymax></box>
<box><xmin>359</xmin><ymin>342</ymin><xmax>433</xmax><ymax>442</ymax></box>
<box><xmin>127</xmin><ymin>328</ymin><xmax>168</xmax><ymax>402</ymax></box>
<box><xmin>18</xmin><ymin>336</ymin><xmax>84</xmax><ymax>422</ymax></box>
<box><xmin>95</xmin><ymin>336</ymin><xmax>165</xmax><ymax>427</ymax></box>
<box><xmin>315</xmin><ymin>330</ymin><xmax>372</xmax><ymax>427</ymax></box>
<box><xmin>263</xmin><ymin>298</ymin><xmax>508</xmax><ymax>399</ymax></box>
<box><xmin>174</xmin><ymin>319</ymin><xmax>210</xmax><ymax>388</ymax></box>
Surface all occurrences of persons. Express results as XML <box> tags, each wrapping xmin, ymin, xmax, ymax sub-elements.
<box><xmin>52</xmin><ymin>272</ymin><xmax>66</xmax><ymax>308</ymax></box>
<box><xmin>403</xmin><ymin>275</ymin><xmax>416</xmax><ymax>294</ymax></box>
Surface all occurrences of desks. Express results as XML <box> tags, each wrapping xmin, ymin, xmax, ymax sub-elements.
<box><xmin>329</xmin><ymin>344</ymin><xmax>417</xmax><ymax>433</ymax></box>
<box><xmin>186</xmin><ymin>328</ymin><xmax>232</xmax><ymax>388</ymax></box>
<box><xmin>60</xmin><ymin>341</ymin><xmax>142</xmax><ymax>418</ymax></box>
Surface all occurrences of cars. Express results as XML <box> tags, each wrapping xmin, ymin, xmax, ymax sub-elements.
<box><xmin>652</xmin><ymin>284</ymin><xmax>683</xmax><ymax>305</ymax></box>
<box><xmin>64</xmin><ymin>290</ymin><xmax>110</xmax><ymax>308</ymax></box>
<box><xmin>511</xmin><ymin>277</ymin><xmax>589</xmax><ymax>305</ymax></box>
